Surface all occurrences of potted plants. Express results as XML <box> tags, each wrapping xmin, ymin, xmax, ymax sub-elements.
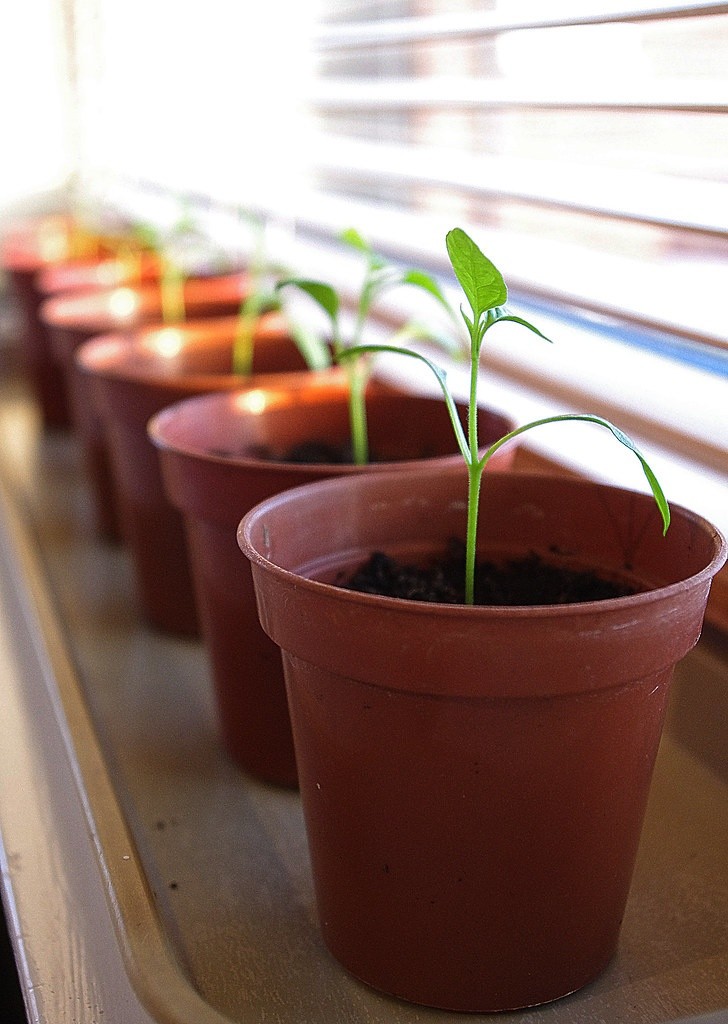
<box><xmin>7</xmin><ymin>217</ymin><xmax>728</xmax><ymax>1014</ymax></box>
